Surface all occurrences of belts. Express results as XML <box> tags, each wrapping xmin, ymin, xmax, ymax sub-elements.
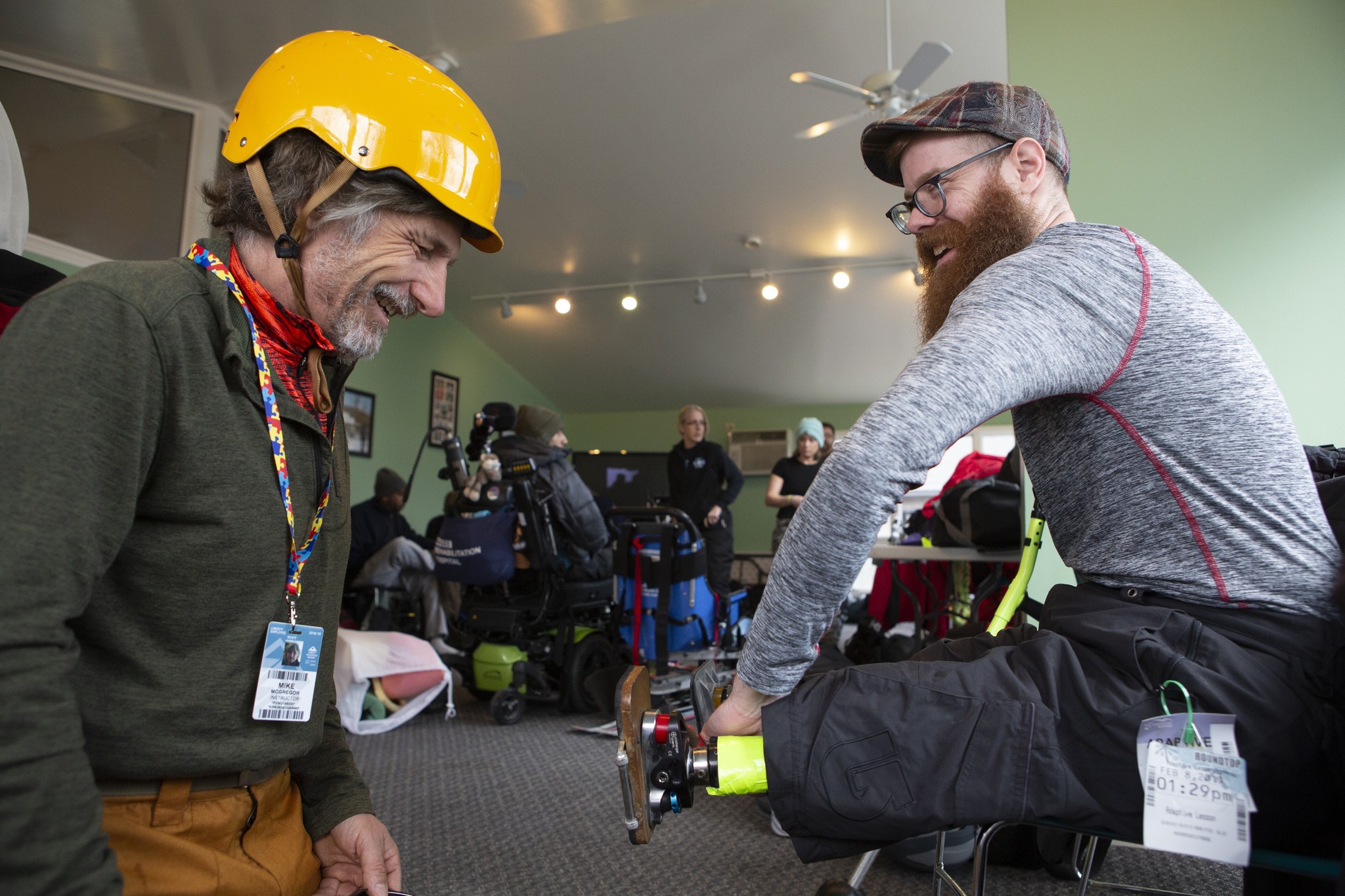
<box><xmin>87</xmin><ymin>761</ymin><xmax>301</xmax><ymax>808</ymax></box>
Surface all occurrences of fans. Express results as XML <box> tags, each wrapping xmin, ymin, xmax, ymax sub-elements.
<box><xmin>788</xmin><ymin>0</ymin><xmax>951</xmax><ymax>140</ymax></box>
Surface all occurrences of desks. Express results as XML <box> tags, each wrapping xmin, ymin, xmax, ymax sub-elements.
<box><xmin>868</xmin><ymin>543</ymin><xmax>1023</xmax><ymax>651</ymax></box>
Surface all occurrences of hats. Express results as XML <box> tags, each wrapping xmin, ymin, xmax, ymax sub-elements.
<box><xmin>514</xmin><ymin>403</ymin><xmax>564</xmax><ymax>447</ymax></box>
<box><xmin>375</xmin><ymin>468</ymin><xmax>407</xmax><ymax>498</ymax></box>
<box><xmin>853</xmin><ymin>75</ymin><xmax>1075</xmax><ymax>193</ymax></box>
<box><xmin>797</xmin><ymin>416</ymin><xmax>827</xmax><ymax>449</ymax></box>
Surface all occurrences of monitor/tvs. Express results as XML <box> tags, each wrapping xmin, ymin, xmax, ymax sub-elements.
<box><xmin>572</xmin><ymin>450</ymin><xmax>673</xmax><ymax>525</ymax></box>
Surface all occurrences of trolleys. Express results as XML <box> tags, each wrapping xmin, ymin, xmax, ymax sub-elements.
<box><xmin>427</xmin><ymin>471</ymin><xmax>629</xmax><ymax>723</ymax></box>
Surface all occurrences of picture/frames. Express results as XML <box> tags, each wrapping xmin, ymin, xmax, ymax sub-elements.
<box><xmin>428</xmin><ymin>369</ymin><xmax>461</xmax><ymax>447</ymax></box>
<box><xmin>342</xmin><ymin>386</ymin><xmax>376</xmax><ymax>458</ymax></box>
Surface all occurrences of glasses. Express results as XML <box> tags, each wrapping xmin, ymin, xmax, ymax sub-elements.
<box><xmin>682</xmin><ymin>419</ymin><xmax>707</xmax><ymax>428</ymax></box>
<box><xmin>823</xmin><ymin>431</ymin><xmax>836</xmax><ymax>440</ymax></box>
<box><xmin>884</xmin><ymin>139</ymin><xmax>1019</xmax><ymax>236</ymax></box>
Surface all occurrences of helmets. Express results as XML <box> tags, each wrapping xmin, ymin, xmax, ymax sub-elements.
<box><xmin>220</xmin><ymin>29</ymin><xmax>505</xmax><ymax>260</ymax></box>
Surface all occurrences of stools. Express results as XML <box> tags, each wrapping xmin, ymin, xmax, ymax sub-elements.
<box><xmin>934</xmin><ymin>820</ymin><xmax>1345</xmax><ymax>895</ymax></box>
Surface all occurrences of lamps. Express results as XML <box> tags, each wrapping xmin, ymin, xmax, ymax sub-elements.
<box><xmin>501</xmin><ymin>298</ymin><xmax>512</xmax><ymax>319</ymax></box>
<box><xmin>695</xmin><ymin>282</ymin><xmax>707</xmax><ymax>304</ymax></box>
<box><xmin>911</xmin><ymin>266</ymin><xmax>925</xmax><ymax>285</ymax></box>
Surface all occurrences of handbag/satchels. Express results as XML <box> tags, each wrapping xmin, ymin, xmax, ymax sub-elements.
<box><xmin>430</xmin><ymin>504</ymin><xmax>519</xmax><ymax>586</ymax></box>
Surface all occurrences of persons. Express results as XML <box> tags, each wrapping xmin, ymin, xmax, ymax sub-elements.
<box><xmin>281</xmin><ymin>642</ymin><xmax>300</xmax><ymax>667</ymax></box>
<box><xmin>613</xmin><ymin>81</ymin><xmax>1344</xmax><ymax>860</ymax></box>
<box><xmin>0</xmin><ymin>30</ymin><xmax>506</xmax><ymax>896</ymax></box>
<box><xmin>490</xmin><ymin>404</ymin><xmax>620</xmax><ymax>585</ymax></box>
<box><xmin>764</xmin><ymin>415</ymin><xmax>827</xmax><ymax>564</ymax></box>
<box><xmin>815</xmin><ymin>422</ymin><xmax>838</xmax><ymax>475</ymax></box>
<box><xmin>341</xmin><ymin>467</ymin><xmax>467</xmax><ymax>660</ymax></box>
<box><xmin>662</xmin><ymin>403</ymin><xmax>747</xmax><ymax>650</ymax></box>
